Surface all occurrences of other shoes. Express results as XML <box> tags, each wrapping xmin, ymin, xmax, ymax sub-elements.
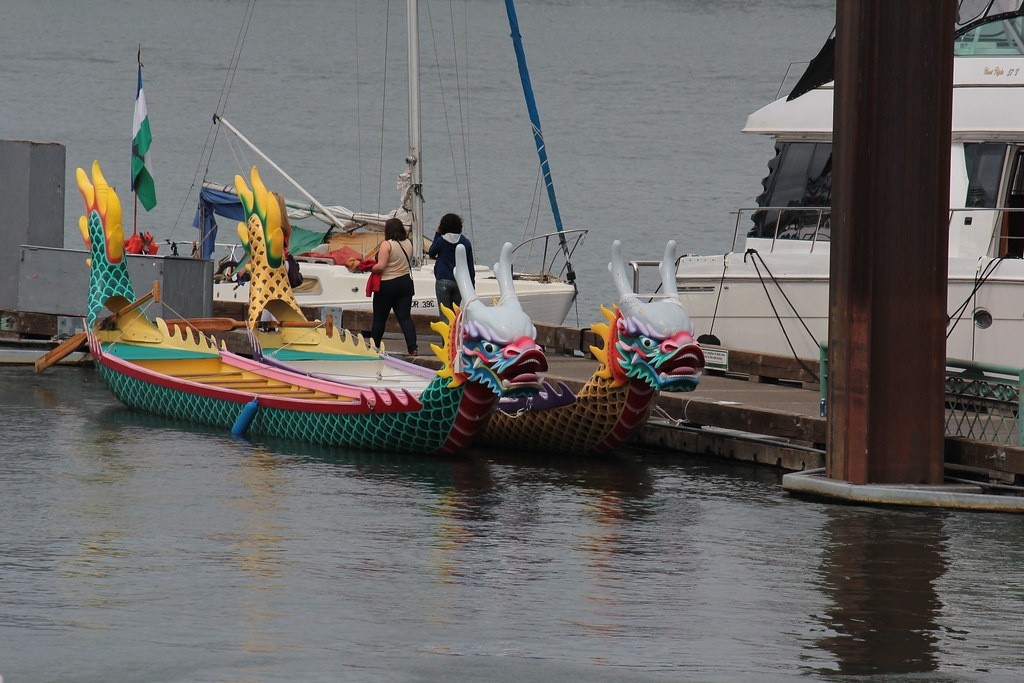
<box><xmin>411</xmin><ymin>349</ymin><xmax>418</xmax><ymax>355</ymax></box>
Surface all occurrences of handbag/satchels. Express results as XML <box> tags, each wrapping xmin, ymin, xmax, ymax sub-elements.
<box><xmin>411</xmin><ymin>279</ymin><xmax>415</xmax><ymax>296</ymax></box>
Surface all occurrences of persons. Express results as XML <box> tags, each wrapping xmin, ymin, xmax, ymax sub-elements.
<box><xmin>369</xmin><ymin>220</ymin><xmax>419</xmax><ymax>357</ymax></box>
<box><xmin>429</xmin><ymin>214</ymin><xmax>476</xmax><ymax>344</ymax></box>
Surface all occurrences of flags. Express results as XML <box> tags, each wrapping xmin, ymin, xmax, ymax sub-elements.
<box><xmin>130</xmin><ymin>49</ymin><xmax>158</xmax><ymax>210</ymax></box>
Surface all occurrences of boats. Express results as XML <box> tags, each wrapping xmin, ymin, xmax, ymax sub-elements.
<box><xmin>74</xmin><ymin>158</ymin><xmax>579</xmax><ymax>460</ymax></box>
<box><xmin>623</xmin><ymin>0</ymin><xmax>1024</xmax><ymax>404</ymax></box>
<box><xmin>231</xmin><ymin>164</ymin><xmax>705</xmax><ymax>460</ymax></box>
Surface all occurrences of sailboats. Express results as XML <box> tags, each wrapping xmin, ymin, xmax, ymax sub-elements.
<box><xmin>154</xmin><ymin>0</ymin><xmax>590</xmax><ymax>336</ymax></box>
